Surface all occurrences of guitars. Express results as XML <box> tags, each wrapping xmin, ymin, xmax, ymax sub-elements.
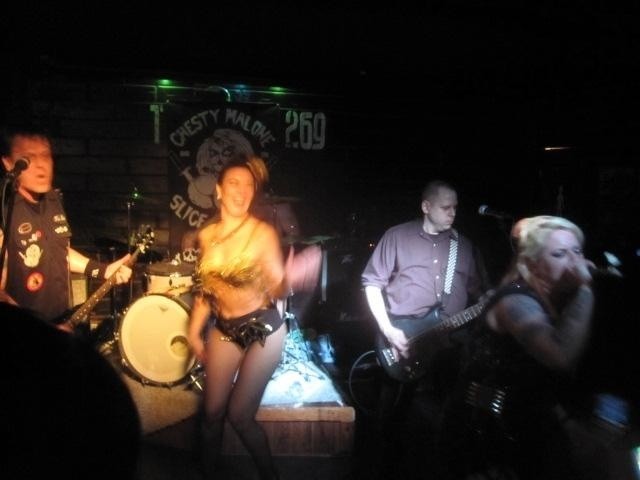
<box><xmin>349</xmin><ymin>302</ymin><xmax>487</xmax><ymax>415</ymax></box>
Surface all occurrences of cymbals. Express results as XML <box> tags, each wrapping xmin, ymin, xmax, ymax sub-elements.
<box><xmin>256</xmin><ymin>198</ymin><xmax>300</xmax><ymax>205</ymax></box>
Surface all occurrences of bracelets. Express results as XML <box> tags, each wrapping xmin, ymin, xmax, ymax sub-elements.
<box><xmin>82</xmin><ymin>259</ymin><xmax>108</xmax><ymax>281</ymax></box>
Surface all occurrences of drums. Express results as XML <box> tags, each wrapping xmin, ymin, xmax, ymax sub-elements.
<box><xmin>145</xmin><ymin>263</ymin><xmax>196</xmax><ymax>299</ymax></box>
<box><xmin>114</xmin><ymin>292</ymin><xmax>196</xmax><ymax>387</ymax></box>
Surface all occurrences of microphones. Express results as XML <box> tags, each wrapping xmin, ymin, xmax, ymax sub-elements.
<box><xmin>571</xmin><ymin>267</ymin><xmax>621</xmax><ymax>279</ymax></box>
<box><xmin>479</xmin><ymin>205</ymin><xmax>504</xmax><ymax>218</ymax></box>
<box><xmin>5</xmin><ymin>158</ymin><xmax>29</xmax><ymax>182</ymax></box>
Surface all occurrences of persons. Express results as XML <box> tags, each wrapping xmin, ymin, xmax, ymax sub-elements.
<box><xmin>441</xmin><ymin>214</ymin><xmax>598</xmax><ymax>479</ymax></box>
<box><xmin>361</xmin><ymin>175</ymin><xmax>499</xmax><ymax>480</ymax></box>
<box><xmin>1</xmin><ymin>117</ymin><xmax>133</xmax><ymax>337</ymax></box>
<box><xmin>185</xmin><ymin>155</ymin><xmax>326</xmax><ymax>478</ymax></box>
